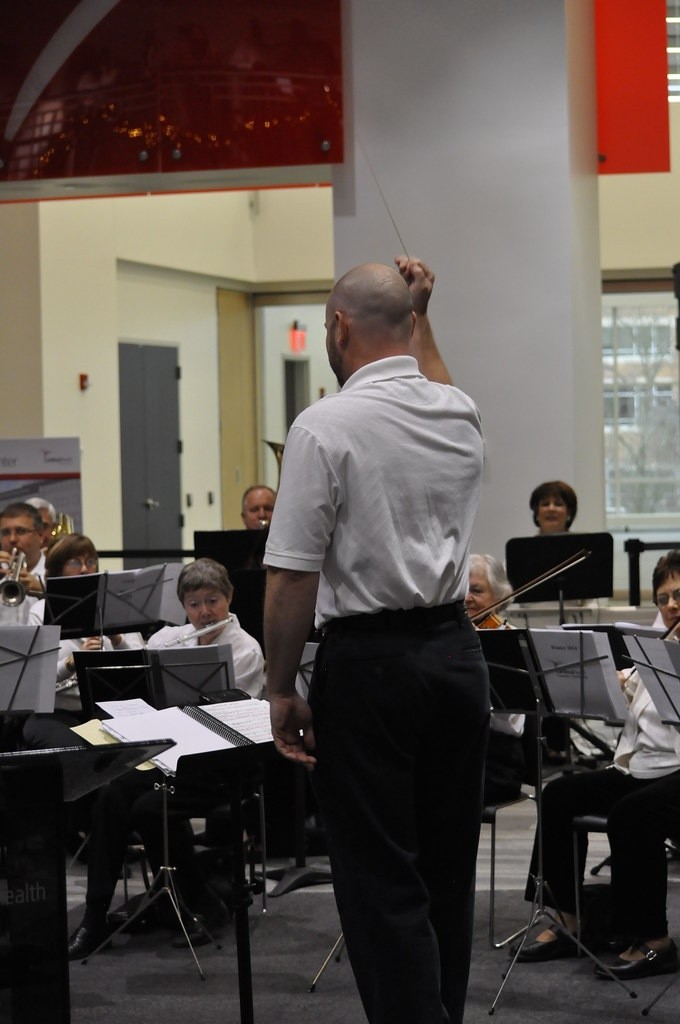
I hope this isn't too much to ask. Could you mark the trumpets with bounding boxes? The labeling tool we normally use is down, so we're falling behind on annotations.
[1,546,28,607]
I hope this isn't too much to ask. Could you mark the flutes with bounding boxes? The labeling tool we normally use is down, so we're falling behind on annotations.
[154,617,235,648]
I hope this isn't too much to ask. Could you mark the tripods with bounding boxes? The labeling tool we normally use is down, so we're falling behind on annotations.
[269,757,335,902]
[479,631,638,1016]
[83,655,229,982]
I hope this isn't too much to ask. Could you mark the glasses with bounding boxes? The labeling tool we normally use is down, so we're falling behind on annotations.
[653,588,680,605]
[0,528,39,536]
[65,557,96,568]
[44,524,53,530]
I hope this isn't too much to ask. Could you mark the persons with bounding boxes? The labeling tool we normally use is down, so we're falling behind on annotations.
[0,478,680,979]
[265,257,480,1024]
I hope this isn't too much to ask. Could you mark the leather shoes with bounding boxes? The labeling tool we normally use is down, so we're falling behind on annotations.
[174,877,254,948]
[68,919,112,961]
[509,924,600,962]
[594,938,680,980]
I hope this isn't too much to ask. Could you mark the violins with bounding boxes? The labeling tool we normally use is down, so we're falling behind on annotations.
[473,614,516,629]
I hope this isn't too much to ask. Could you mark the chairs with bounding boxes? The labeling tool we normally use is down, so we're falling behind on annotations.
[112,793,260,933]
[573,816,613,896]
[466,628,547,949]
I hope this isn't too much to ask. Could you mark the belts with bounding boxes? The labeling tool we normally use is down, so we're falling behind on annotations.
[316,599,468,634]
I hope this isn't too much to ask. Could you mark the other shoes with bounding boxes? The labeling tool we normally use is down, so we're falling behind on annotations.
[118,848,145,878]
